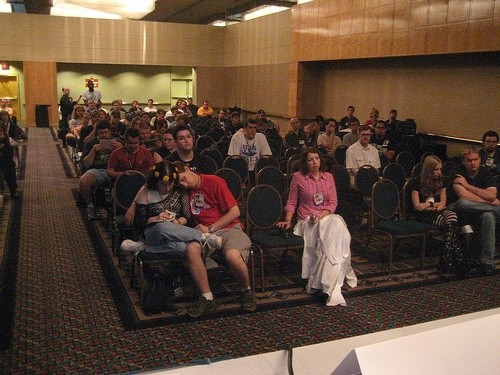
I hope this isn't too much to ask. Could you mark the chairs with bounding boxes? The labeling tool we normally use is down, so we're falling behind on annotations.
[71,115,500,308]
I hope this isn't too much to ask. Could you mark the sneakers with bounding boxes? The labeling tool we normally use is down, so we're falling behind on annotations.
[202,232,223,251]
[186,295,216,318]
[120,239,145,256]
[87,205,97,221]
[239,289,256,312]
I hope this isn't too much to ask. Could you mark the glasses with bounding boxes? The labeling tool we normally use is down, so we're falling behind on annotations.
[360,134,371,137]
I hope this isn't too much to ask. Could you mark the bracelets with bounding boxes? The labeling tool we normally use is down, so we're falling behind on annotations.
[208,225,214,233]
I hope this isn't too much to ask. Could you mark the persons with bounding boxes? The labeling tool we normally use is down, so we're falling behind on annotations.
[163,126,213,175]
[124,162,256,316]
[228,119,272,171]
[138,120,162,154]
[412,156,468,278]
[449,151,500,276]
[288,106,399,226]
[216,105,275,133]
[0,99,22,206]
[80,121,123,221]
[276,147,357,308]
[120,166,222,253]
[108,129,153,177]
[478,130,500,175]
[59,81,212,134]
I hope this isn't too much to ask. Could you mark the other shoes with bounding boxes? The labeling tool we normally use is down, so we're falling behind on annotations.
[442,266,457,279]
[482,264,494,275]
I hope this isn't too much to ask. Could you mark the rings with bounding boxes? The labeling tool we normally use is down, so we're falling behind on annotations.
[163,218,165,220]
